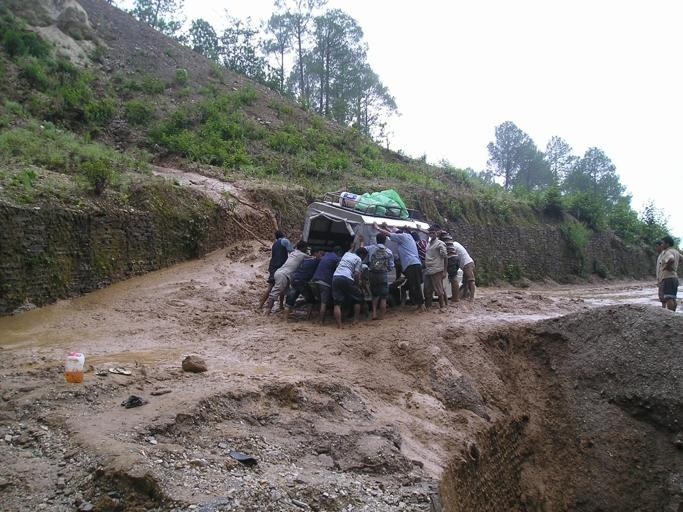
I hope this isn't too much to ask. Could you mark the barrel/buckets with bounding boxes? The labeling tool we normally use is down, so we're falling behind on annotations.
[65,352,85,383]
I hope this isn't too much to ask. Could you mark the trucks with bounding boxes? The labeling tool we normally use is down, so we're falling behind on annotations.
[301,192,476,308]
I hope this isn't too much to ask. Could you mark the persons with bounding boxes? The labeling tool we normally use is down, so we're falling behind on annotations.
[253,220,477,331]
[658,234,683,313]
[653,241,673,308]
